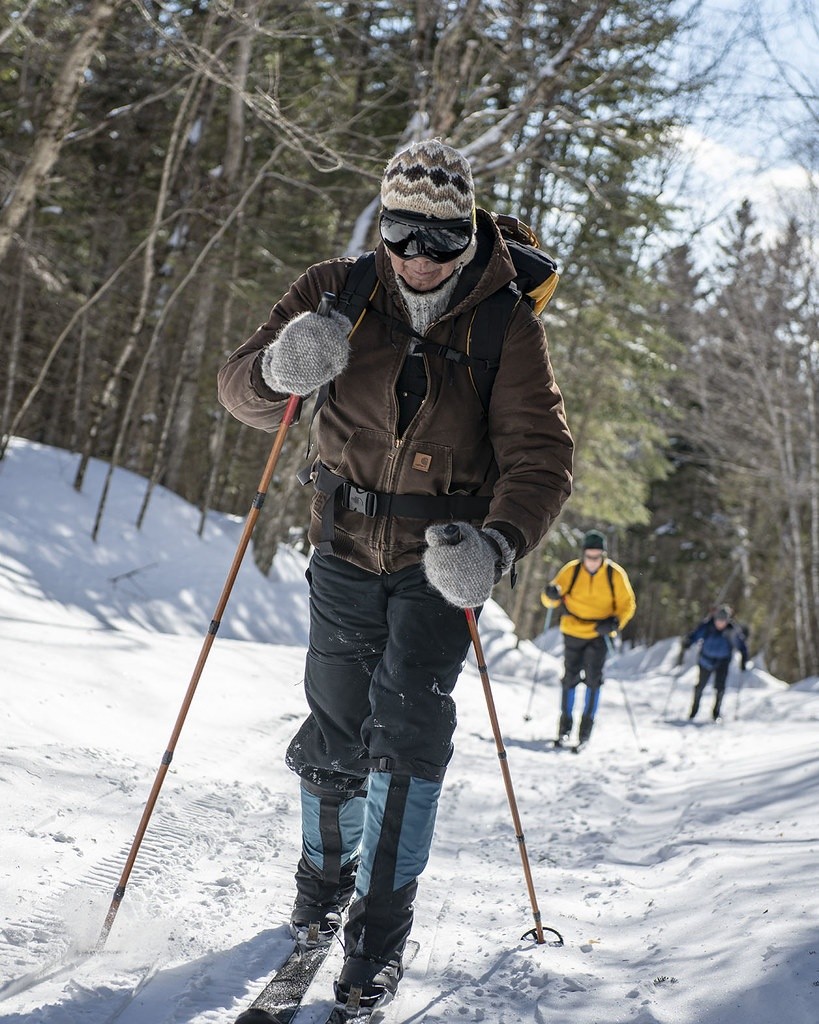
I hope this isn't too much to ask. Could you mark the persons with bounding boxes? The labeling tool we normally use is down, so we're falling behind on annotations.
[681,603,750,721]
[217,140,574,1013]
[541,531,637,744]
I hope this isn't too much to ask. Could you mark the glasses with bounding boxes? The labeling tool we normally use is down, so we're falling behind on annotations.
[379,209,473,264]
[584,553,601,560]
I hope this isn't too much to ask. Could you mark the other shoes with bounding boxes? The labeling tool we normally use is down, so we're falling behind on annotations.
[291,901,345,943]
[336,951,401,1009]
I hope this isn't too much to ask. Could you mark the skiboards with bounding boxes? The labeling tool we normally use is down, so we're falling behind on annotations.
[234,939,335,1024]
[324,938,421,1024]
[554,736,588,754]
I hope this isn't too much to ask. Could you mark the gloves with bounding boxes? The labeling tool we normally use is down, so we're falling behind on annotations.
[595,615,620,637]
[545,583,561,601]
[262,309,353,396]
[682,637,691,649]
[423,521,515,608]
[741,660,747,672]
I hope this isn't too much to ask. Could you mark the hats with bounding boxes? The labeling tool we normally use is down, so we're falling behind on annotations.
[582,530,607,551]
[714,605,731,620]
[381,139,473,220]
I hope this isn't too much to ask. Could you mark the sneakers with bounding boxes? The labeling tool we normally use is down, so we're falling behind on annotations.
[559,715,573,734]
[579,717,594,741]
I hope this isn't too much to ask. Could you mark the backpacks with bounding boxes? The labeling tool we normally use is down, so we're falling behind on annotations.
[558,560,616,625]
[295,210,559,556]
